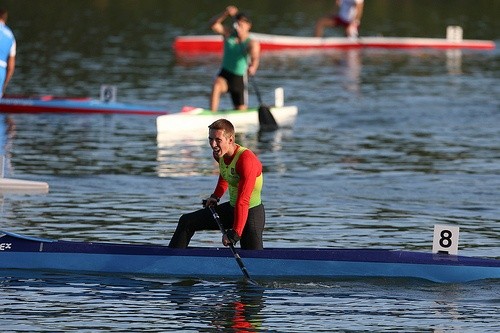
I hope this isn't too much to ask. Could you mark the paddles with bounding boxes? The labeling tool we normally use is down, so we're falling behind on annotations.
[231,14,279,132]
[201,197,262,287]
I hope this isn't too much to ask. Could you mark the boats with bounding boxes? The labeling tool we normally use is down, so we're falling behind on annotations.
[156,86,298,135]
[0,84,166,121]
[173,25,496,58]
[0,229,500,284]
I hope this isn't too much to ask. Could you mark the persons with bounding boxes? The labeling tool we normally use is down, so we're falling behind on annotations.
[210,5,260,111]
[316,0,364,37]
[168,119,265,248]
[0,7,16,98]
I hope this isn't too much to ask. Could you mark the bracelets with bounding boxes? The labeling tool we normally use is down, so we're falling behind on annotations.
[210,194,220,202]
